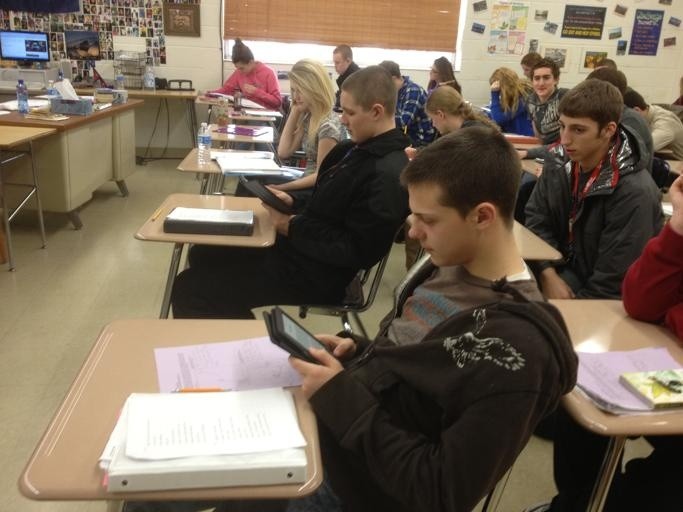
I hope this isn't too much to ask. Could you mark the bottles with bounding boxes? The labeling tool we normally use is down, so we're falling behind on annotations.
[47,79,57,113]
[233,88,242,111]
[144,65,155,91]
[217,96,229,128]
[115,70,124,90]
[57,67,64,81]
[196,122,211,164]
[16,79,29,114]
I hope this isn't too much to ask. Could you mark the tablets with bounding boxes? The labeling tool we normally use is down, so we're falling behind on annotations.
[238,173,290,213]
[272,307,336,365]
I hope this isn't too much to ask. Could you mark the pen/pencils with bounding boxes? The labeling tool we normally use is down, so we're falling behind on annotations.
[151,209,163,221]
[171,387,231,392]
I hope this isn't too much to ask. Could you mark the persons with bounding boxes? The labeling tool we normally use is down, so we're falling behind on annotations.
[532,173,683,512]
[206,38,282,110]
[235,59,347,200]
[171,64,414,323]
[333,46,683,170]
[288,125,579,512]
[521,79,662,298]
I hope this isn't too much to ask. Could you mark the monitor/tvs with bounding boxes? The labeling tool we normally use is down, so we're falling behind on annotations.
[0,30,50,68]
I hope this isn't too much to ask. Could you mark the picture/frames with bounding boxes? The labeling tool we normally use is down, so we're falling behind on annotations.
[162,2,201,37]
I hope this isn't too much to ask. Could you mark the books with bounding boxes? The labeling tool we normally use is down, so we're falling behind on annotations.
[99,393,308,494]
[164,207,254,237]
